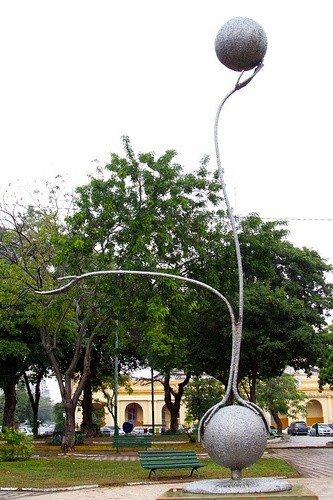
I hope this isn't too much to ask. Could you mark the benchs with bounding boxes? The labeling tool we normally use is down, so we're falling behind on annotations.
[112,435,153,454]
[138,449,207,480]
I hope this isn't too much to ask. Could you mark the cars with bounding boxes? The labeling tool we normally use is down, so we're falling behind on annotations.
[270,425,276,433]
[310,423,333,436]
[0,423,56,437]
[100,423,195,436]
[286,421,308,436]
